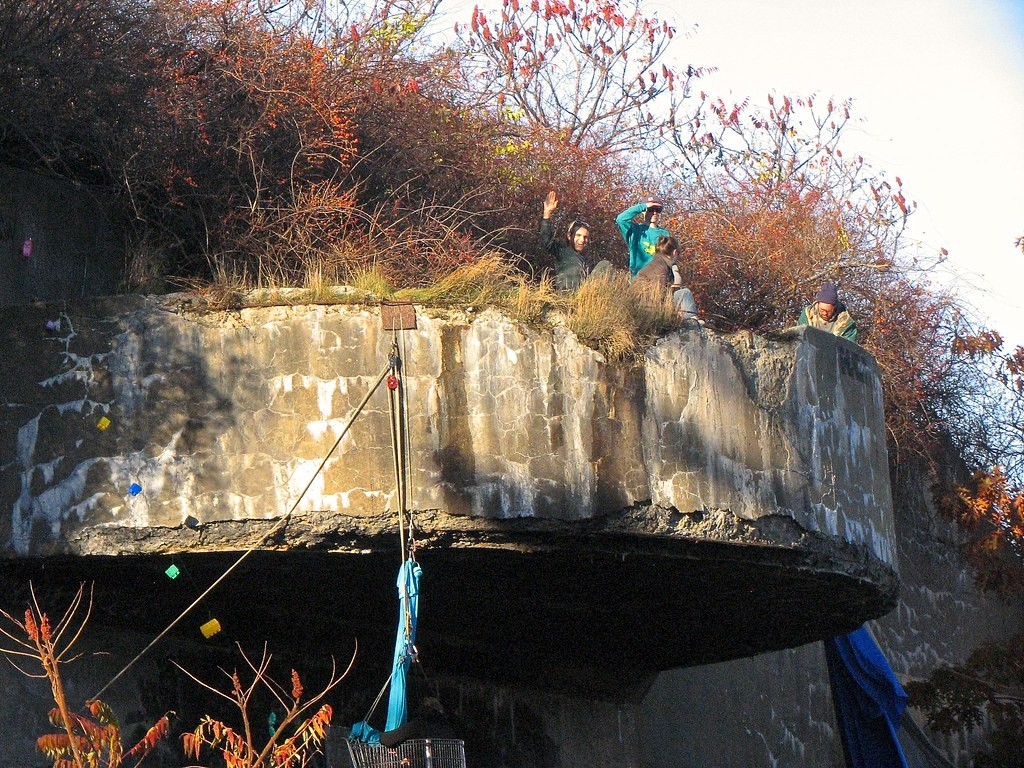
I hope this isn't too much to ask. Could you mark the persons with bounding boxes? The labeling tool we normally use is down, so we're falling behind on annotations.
[629,235,705,327]
[541,191,591,298]
[798,283,858,343]
[615,195,678,284]
[269,693,318,768]
[379,698,458,768]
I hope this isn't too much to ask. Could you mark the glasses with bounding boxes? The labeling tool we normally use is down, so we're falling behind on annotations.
[568,220,590,233]
[648,207,662,212]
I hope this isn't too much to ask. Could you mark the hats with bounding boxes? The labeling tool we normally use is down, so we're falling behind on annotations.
[642,195,663,205]
[817,283,837,304]
